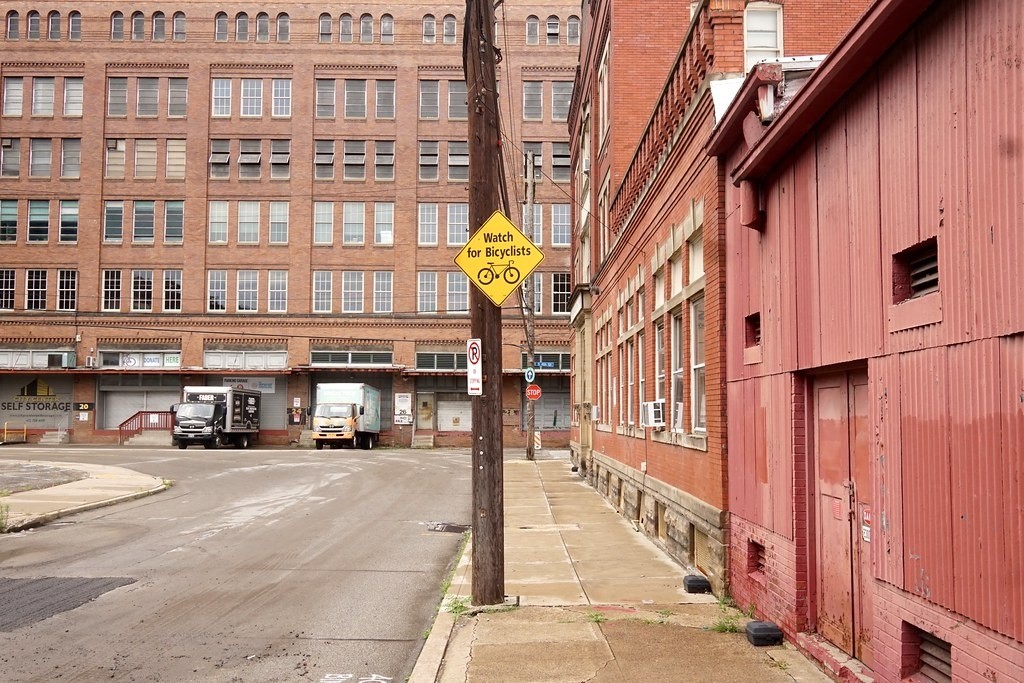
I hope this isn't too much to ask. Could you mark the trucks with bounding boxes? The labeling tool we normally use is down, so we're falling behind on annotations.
[169,385,261,449]
[307,382,382,450]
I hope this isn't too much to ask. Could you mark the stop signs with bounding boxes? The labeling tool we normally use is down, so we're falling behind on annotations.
[526,384,542,400]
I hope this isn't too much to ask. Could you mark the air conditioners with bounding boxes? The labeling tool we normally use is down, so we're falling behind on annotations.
[47,353,76,367]
[591,406,600,421]
[86,356,100,369]
[641,402,666,427]
